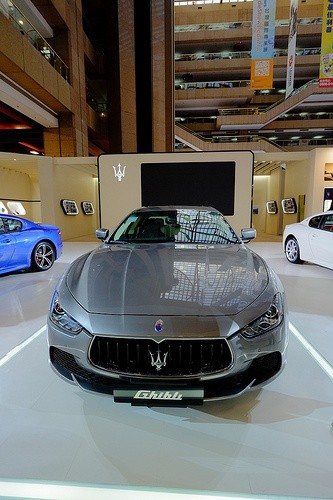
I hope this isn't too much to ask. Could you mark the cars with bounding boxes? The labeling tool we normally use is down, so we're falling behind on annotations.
[0,214,64,276]
[45,205,289,408]
[282,211,333,270]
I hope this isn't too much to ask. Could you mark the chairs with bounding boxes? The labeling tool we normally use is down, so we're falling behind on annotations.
[142,218,164,239]
